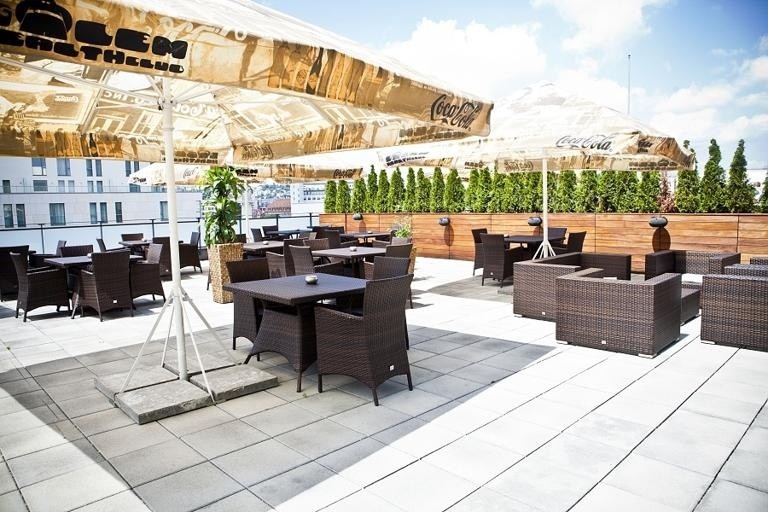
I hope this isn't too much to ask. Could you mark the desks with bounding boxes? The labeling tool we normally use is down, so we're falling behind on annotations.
[221,273,371,392]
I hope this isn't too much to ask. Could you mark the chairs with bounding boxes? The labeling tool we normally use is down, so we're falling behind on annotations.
[2,231,201,322]
[314,274,415,405]
[471,227,587,289]
[227,226,414,361]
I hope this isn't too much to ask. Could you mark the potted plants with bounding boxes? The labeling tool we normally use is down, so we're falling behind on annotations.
[202,168,244,303]
[318,137,768,273]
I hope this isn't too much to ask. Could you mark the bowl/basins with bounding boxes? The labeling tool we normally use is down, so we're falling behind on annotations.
[305,276,318,285]
[349,246,358,252]
[263,241,269,245]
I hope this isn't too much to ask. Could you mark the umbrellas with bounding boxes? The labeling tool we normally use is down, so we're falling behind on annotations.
[127,155,369,242]
[0,0,494,383]
[376,79,696,262]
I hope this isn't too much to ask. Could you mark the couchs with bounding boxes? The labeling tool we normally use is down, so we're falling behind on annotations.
[514,251,768,359]
